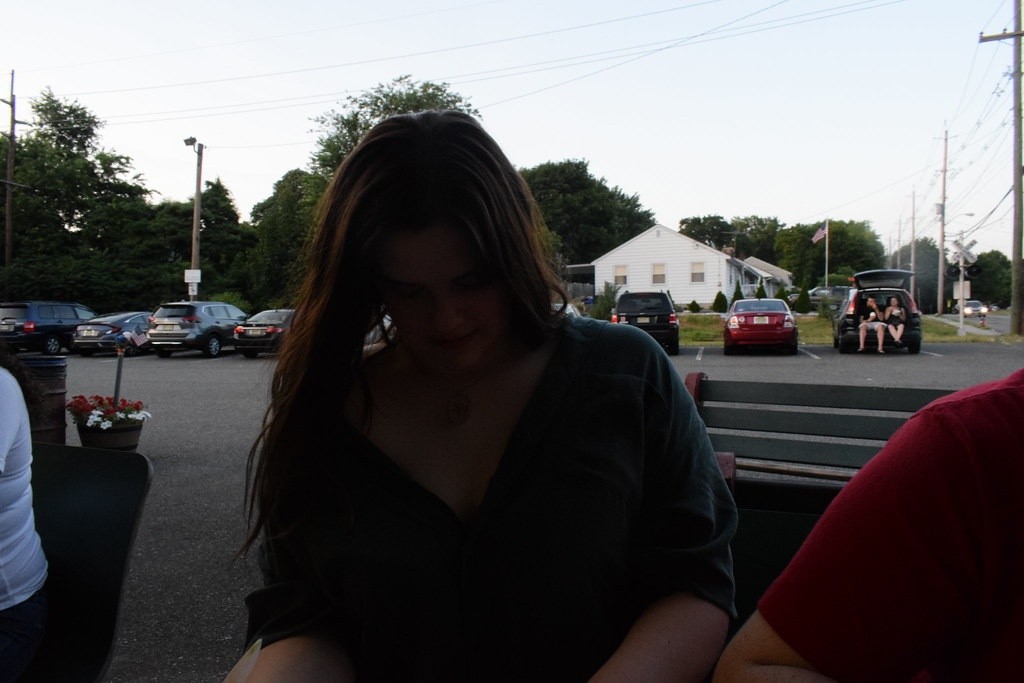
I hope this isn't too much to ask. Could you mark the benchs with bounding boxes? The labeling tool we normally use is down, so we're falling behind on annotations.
[684,371,960,640]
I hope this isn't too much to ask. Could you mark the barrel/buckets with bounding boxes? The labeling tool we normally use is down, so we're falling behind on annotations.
[2,355,68,448]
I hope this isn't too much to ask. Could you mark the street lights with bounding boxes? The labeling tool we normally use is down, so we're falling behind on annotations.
[936,212,976,315]
[184,136,207,300]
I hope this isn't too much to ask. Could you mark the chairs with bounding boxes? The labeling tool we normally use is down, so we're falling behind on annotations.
[16,441,152,681]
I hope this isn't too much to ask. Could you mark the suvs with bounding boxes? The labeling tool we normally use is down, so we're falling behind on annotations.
[830,268,923,355]
[146,299,251,358]
[0,300,100,355]
[610,289,680,356]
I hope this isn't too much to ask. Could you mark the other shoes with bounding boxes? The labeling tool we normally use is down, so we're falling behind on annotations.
[893,341,905,348]
[857,348,865,352]
[877,349,885,353]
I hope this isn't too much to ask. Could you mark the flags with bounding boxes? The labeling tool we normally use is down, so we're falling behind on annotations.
[131,325,147,346]
[811,222,826,243]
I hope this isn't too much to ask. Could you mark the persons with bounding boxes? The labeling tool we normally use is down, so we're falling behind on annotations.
[225,110,739,683]
[0,347,48,683]
[857,294,886,353]
[885,296,906,343]
[713,366,1024,683]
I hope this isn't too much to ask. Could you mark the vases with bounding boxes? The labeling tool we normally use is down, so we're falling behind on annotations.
[77,414,142,454]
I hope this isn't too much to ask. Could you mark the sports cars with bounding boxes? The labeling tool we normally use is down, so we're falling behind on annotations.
[71,310,156,358]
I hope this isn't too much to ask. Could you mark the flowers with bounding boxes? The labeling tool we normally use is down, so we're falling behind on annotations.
[70,393,153,430]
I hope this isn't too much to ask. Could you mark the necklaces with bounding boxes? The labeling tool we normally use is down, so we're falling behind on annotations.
[419,361,474,423]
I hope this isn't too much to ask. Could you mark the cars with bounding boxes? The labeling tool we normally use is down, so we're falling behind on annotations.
[553,302,582,320]
[788,286,846,313]
[951,299,1001,318]
[720,298,798,356]
[233,309,297,358]
